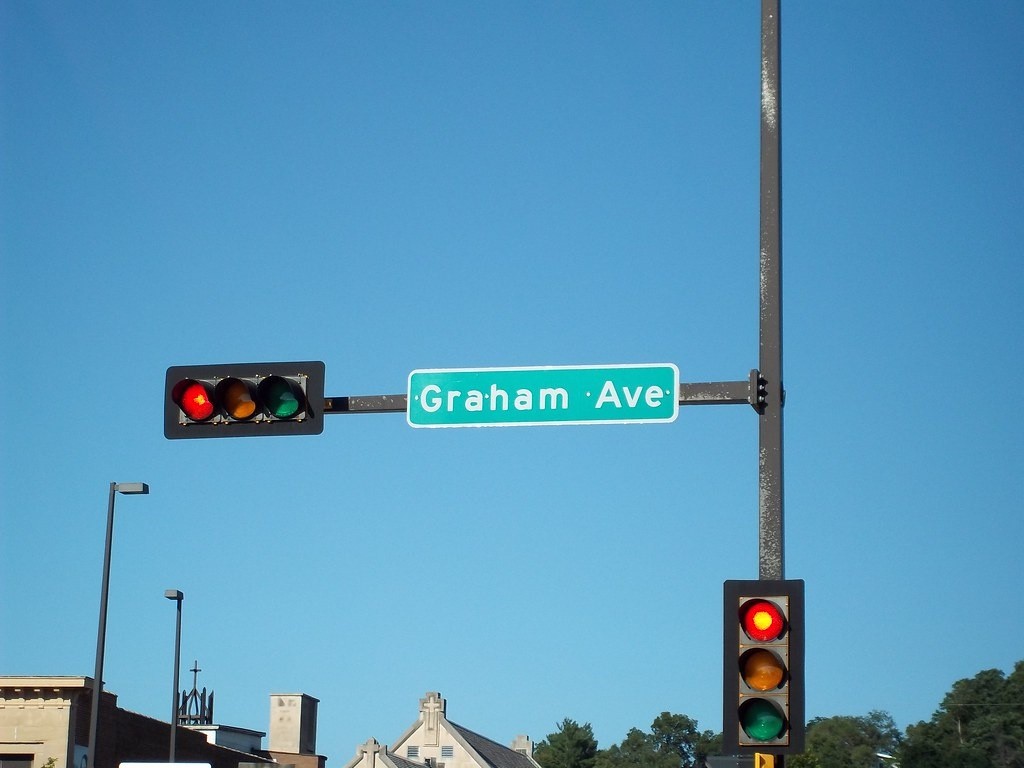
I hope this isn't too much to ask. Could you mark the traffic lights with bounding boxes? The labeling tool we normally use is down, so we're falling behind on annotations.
[724,579,807,755]
[163,361,325,438]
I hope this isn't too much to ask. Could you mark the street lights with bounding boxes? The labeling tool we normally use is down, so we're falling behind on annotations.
[85,480,150,768]
[164,589,185,762]
